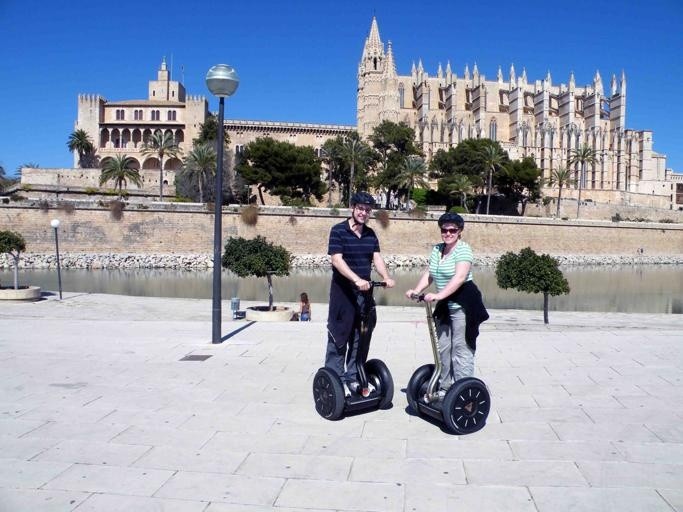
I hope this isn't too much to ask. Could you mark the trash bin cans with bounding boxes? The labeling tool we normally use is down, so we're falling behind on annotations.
[231,298,240,319]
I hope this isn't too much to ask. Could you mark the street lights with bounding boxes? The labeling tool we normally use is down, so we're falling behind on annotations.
[199,60,239,342]
[50,218,63,298]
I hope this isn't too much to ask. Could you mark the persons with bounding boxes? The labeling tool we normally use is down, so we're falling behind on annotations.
[295,292,311,322]
[406,210,489,402]
[323,191,395,395]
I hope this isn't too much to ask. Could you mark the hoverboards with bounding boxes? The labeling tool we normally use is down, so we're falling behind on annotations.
[310,280,396,421]
[406,289,493,433]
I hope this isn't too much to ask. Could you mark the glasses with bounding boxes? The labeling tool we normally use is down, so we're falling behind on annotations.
[358,207,372,214]
[441,227,460,234]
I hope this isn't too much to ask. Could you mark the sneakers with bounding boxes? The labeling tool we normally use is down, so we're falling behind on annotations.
[351,382,376,392]
[344,384,352,398]
[437,390,447,397]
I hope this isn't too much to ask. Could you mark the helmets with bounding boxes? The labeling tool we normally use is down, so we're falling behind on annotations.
[438,213,464,228]
[351,192,374,205]
